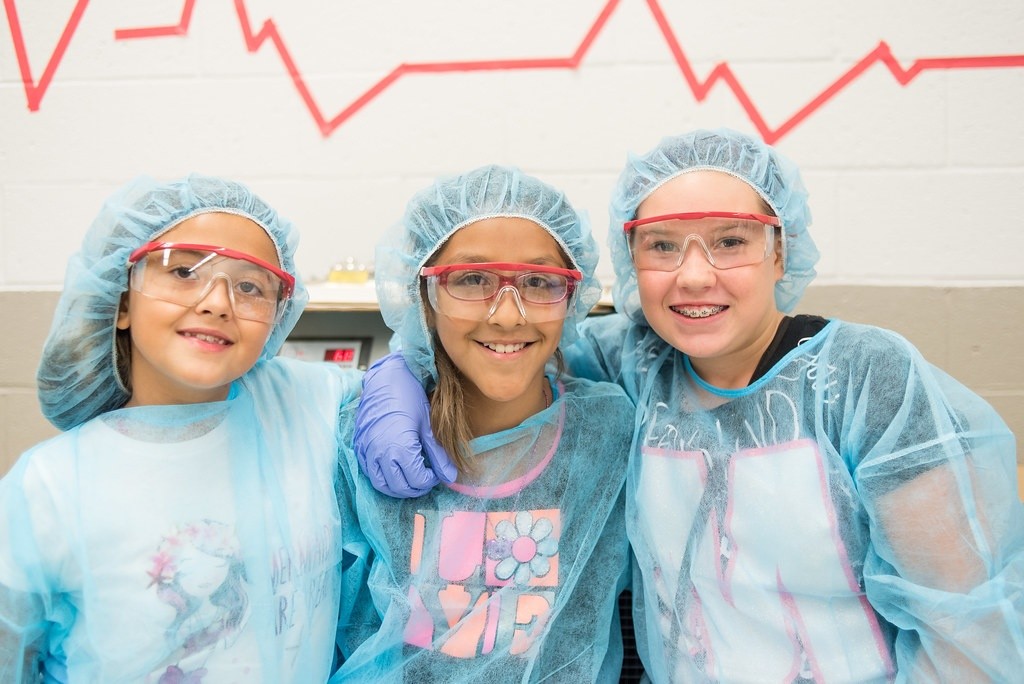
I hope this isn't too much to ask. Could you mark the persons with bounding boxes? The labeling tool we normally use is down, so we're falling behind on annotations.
[0,172,367,684]
[353,126,1024,684]
[331,167,635,684]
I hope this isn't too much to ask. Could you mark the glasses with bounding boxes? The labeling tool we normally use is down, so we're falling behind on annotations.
[419,262,584,323]
[128,241,295,325]
[623,211,780,271]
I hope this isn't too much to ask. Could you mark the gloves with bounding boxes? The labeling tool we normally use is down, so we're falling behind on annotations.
[353,350,457,498]
